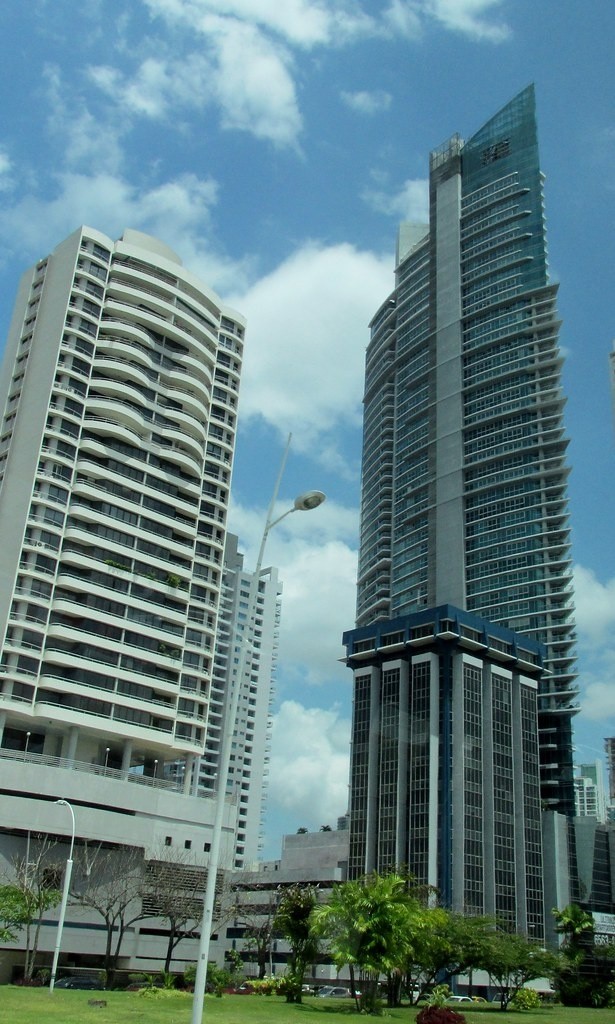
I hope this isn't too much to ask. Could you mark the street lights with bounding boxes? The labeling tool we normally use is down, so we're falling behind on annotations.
[189,428,336,1024]
[47,798,75,989]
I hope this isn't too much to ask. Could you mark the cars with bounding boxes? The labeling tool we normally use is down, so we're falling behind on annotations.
[127,983,161,992]
[216,979,559,1005]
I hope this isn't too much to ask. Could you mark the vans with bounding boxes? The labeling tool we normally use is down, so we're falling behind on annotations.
[55,976,104,992]
